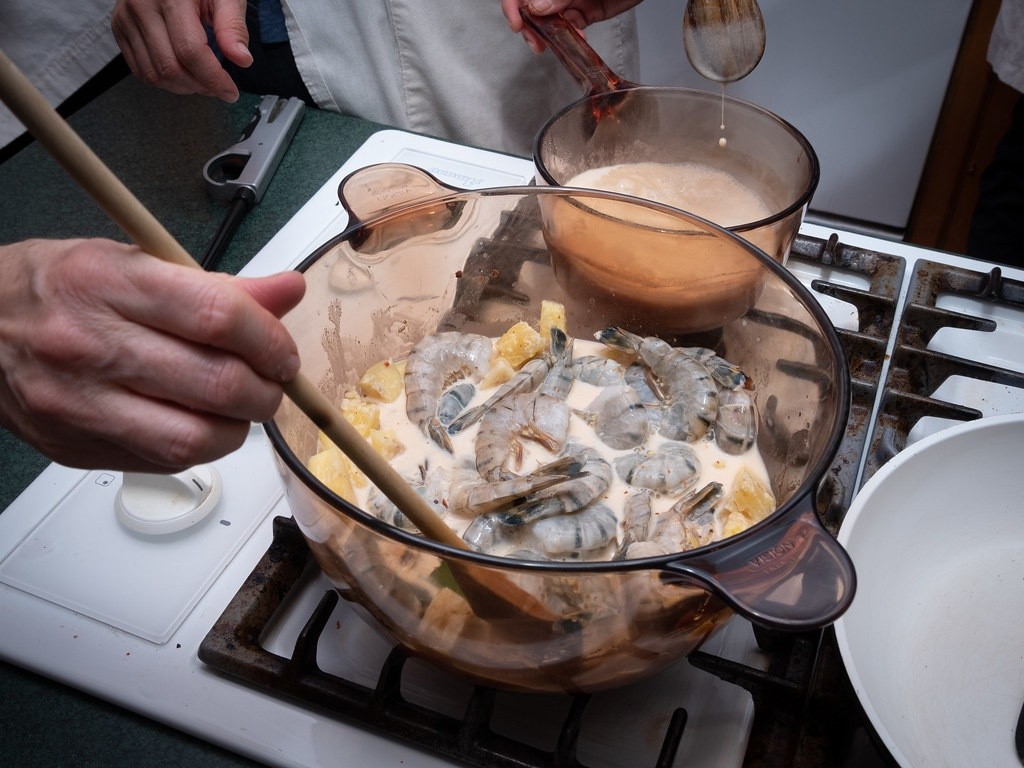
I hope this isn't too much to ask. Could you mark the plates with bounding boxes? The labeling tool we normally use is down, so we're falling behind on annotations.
[832,410,1024,768]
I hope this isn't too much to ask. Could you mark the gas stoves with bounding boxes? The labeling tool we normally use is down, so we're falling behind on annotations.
[0,129,1024,768]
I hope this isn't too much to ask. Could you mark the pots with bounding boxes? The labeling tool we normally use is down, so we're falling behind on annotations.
[518,0,821,331]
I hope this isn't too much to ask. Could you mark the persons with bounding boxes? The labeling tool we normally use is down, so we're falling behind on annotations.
[499,0,1024,277]
[106,0,651,169]
[0,0,311,480]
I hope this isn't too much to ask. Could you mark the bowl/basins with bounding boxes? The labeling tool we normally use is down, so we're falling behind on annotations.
[260,161,857,695]
